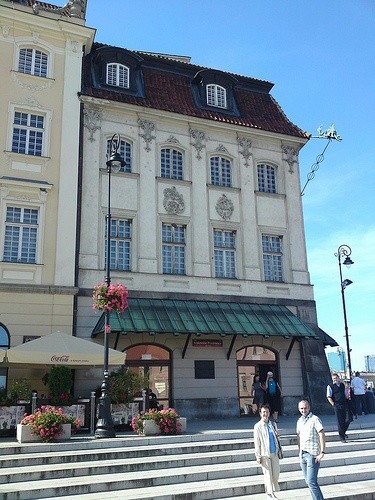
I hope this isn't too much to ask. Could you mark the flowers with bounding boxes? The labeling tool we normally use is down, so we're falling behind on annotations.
[21,405,81,443]
[92,281,129,313]
[132,407,182,436]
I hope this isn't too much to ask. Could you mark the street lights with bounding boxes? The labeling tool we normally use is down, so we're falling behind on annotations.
[94,131,128,438]
[333,244,355,400]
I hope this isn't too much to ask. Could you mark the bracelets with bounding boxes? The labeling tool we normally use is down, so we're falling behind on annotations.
[321,451,325,454]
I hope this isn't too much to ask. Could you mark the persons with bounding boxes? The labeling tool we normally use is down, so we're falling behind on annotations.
[327,373,352,443]
[134,389,161,413]
[351,371,368,415]
[251,375,265,416]
[296,400,326,500]
[365,387,375,414]
[30,390,42,413]
[254,405,283,500]
[263,371,283,423]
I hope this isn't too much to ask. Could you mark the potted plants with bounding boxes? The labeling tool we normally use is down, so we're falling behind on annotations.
[110,366,152,426]
[0,365,86,439]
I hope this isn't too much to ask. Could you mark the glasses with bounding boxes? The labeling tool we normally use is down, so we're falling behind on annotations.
[269,376,273,378]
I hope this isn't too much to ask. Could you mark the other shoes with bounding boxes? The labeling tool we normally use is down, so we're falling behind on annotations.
[341,438,346,442]
[345,433,349,439]
[272,493,279,500]
[272,418,279,423]
[267,493,274,500]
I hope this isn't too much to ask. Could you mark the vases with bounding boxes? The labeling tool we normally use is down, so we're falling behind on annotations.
[16,424,71,444]
[142,417,187,436]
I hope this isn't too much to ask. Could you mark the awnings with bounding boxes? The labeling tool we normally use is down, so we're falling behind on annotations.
[93,296,319,361]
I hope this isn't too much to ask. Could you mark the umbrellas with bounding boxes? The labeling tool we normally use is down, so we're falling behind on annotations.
[5,330,126,364]
[0,348,6,364]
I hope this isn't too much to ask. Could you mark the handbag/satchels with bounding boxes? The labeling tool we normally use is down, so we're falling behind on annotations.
[278,442,284,459]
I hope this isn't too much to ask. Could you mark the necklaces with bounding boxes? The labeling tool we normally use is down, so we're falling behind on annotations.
[269,380,272,385]
